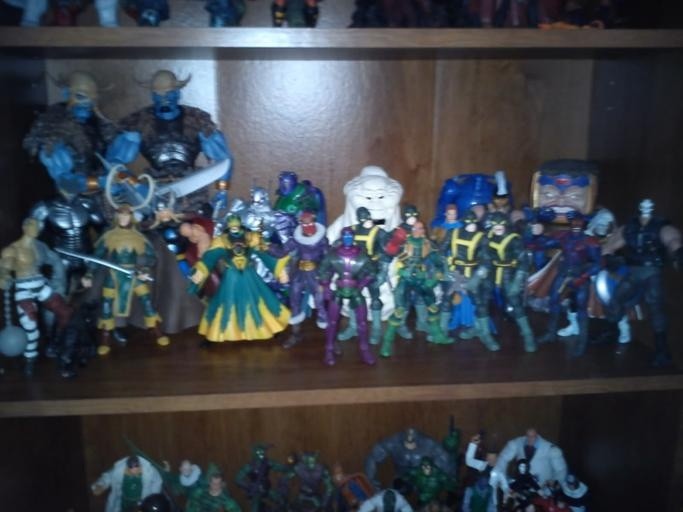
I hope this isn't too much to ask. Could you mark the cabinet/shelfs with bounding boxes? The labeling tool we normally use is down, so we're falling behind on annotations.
[0,19,683,419]
